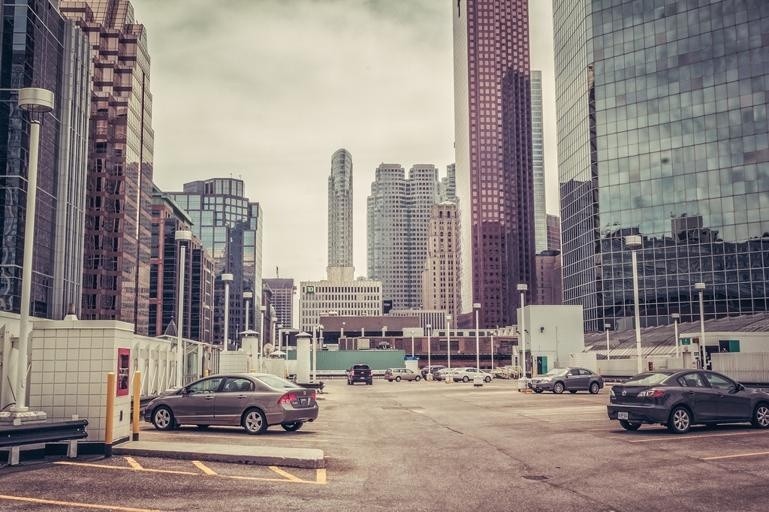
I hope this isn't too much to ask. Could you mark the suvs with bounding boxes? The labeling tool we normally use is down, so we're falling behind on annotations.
[345,364,373,385]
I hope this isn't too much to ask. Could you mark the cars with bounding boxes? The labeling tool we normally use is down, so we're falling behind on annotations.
[420,364,521,383]
[142,371,319,435]
[606,369,769,434]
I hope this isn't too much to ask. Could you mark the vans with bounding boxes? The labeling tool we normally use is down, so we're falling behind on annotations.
[384,368,423,382]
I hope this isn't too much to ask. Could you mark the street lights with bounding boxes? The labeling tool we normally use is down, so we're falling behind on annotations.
[221,271,233,351]
[473,302,484,386]
[516,283,530,394]
[241,290,289,360]
[622,234,644,376]
[311,324,321,381]
[489,329,494,372]
[527,366,603,395]
[693,282,707,370]
[670,312,680,359]
[411,315,458,383]
[5,82,59,421]
[603,323,612,359]
[173,229,194,390]
